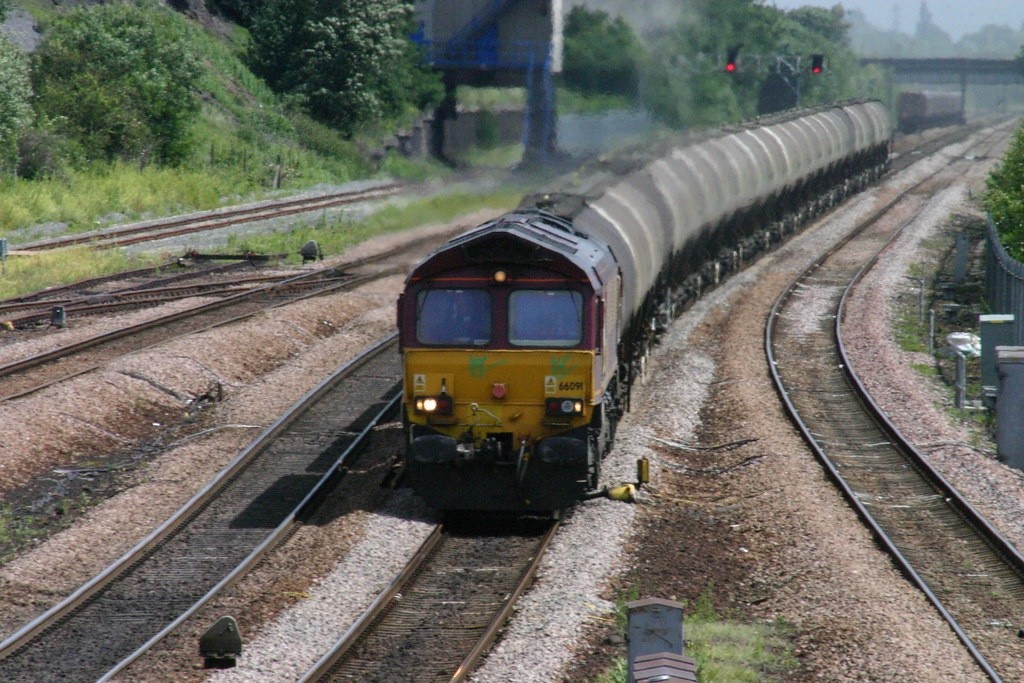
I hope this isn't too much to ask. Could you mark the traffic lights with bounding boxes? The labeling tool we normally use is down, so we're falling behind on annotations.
[813,54,823,74]
[726,48,739,73]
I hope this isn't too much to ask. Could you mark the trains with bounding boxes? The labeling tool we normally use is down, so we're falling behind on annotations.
[897,91,966,129]
[395,98,893,511]
